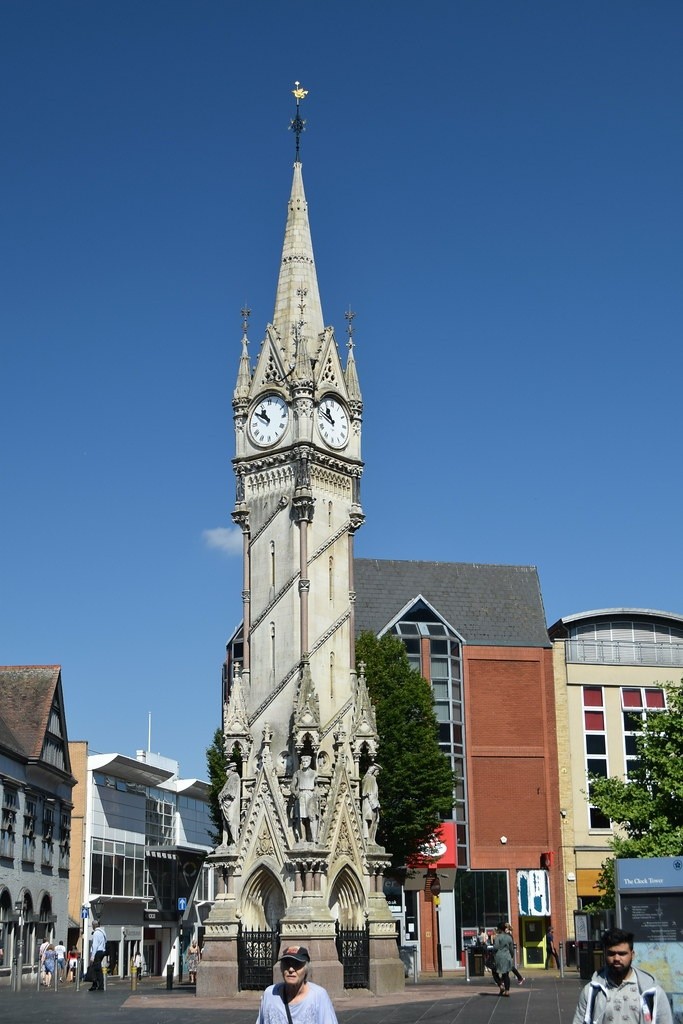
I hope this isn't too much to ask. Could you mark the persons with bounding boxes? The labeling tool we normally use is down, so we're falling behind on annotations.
[255,945,339,1024]
[185,939,204,983]
[475,922,562,997]
[40,920,143,992]
[572,926,673,1024]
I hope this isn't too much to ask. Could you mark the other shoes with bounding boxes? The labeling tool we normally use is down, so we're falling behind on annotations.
[97,987,104,991]
[517,977,525,986]
[60,977,63,983]
[498,989,505,996]
[88,987,97,991]
[503,994,510,997]
[494,980,504,986]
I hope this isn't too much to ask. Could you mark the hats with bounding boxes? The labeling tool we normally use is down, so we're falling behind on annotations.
[278,946,310,963]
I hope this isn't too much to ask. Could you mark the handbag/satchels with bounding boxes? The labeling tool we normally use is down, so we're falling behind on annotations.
[485,955,496,969]
[83,966,94,982]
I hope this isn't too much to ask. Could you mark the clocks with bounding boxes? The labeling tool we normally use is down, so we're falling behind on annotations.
[315,396,351,450]
[246,393,289,450]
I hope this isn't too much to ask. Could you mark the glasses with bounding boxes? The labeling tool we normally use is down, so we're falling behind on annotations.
[281,960,305,970]
